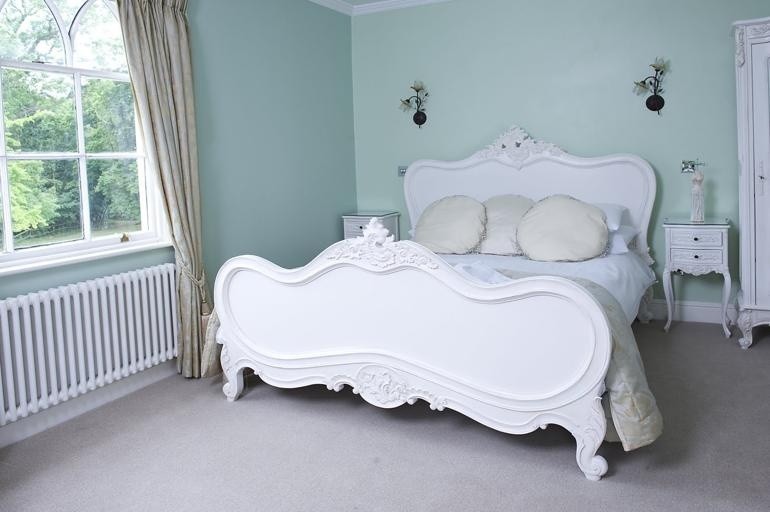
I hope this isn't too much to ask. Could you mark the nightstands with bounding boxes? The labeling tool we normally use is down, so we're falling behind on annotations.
[342,211,401,243]
[661,216,731,338]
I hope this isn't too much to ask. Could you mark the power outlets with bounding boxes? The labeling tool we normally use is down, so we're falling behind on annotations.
[398,166,408,176]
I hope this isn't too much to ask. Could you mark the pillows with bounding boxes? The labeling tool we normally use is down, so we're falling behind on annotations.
[608,225,641,254]
[474,194,533,256]
[592,201,626,233]
[517,193,608,262]
[414,195,487,256]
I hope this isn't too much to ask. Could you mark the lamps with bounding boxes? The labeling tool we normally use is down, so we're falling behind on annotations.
[633,57,668,111]
[399,81,429,128]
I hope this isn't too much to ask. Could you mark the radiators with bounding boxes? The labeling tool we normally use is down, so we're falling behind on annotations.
[1,263,180,427]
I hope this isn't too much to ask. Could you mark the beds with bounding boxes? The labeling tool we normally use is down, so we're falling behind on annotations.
[214,125,664,481]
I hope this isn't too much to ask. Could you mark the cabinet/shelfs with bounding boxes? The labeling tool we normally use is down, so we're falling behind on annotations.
[750,42,770,309]
